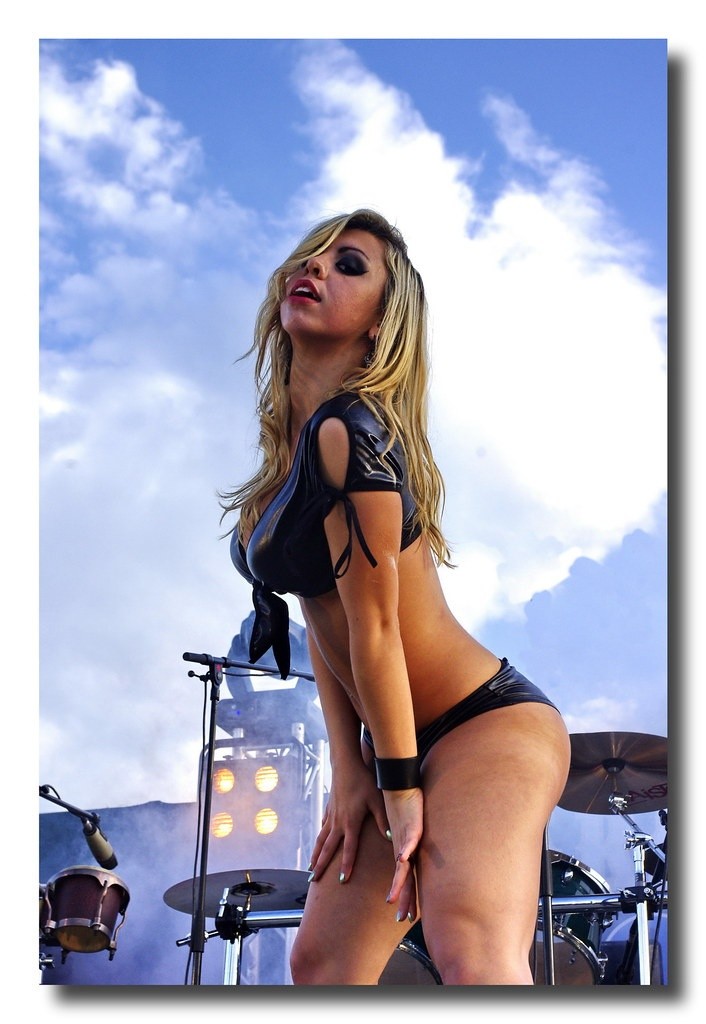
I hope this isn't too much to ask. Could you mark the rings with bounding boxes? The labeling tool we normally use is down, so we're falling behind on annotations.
[395,856,399,863]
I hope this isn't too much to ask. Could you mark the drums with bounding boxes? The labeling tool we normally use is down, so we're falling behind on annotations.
[528,849,614,985]
[378,919,441,985]
[39,865,130,953]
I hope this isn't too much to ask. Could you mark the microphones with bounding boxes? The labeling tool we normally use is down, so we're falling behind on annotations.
[81,819,118,871]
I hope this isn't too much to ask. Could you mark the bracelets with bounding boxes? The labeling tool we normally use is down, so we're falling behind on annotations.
[375,754,421,791]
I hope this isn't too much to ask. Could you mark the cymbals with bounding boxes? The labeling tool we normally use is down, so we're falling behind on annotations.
[554,730,668,815]
[162,868,312,919]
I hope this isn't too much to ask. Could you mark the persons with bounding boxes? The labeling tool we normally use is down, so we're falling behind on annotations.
[220,209,571,986]
